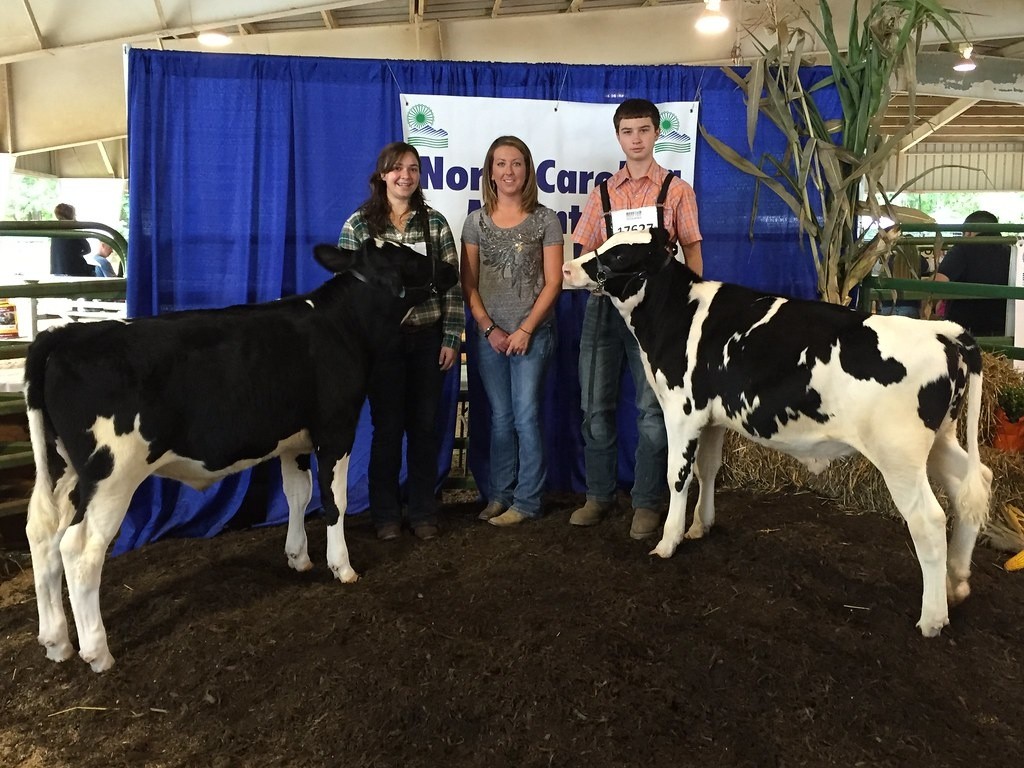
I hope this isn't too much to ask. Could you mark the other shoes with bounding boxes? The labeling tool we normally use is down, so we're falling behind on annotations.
[377,522,401,540]
[478,501,506,521]
[489,505,530,526]
[417,522,438,539]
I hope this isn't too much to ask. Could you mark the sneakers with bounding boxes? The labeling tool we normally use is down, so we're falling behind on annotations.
[569,494,622,526]
[630,504,661,540]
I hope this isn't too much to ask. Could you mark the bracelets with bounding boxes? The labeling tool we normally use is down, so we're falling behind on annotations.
[520,328,532,335]
[484,323,498,339]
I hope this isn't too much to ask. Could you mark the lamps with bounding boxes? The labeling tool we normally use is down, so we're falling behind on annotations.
[694,0,729,35]
[197,27,232,47]
[953,43,976,72]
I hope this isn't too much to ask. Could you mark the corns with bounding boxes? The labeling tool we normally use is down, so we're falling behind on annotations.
[842,223,902,291]
[1004,549,1024,571]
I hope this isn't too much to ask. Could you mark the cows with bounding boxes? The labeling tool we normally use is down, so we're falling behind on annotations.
[26,240,463,676]
[561,226,994,638]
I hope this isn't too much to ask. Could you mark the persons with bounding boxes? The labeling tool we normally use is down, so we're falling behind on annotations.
[50,203,118,312]
[874,211,1011,337]
[569,98,704,539]
[334,142,465,539]
[95,241,123,277]
[460,135,564,527]
